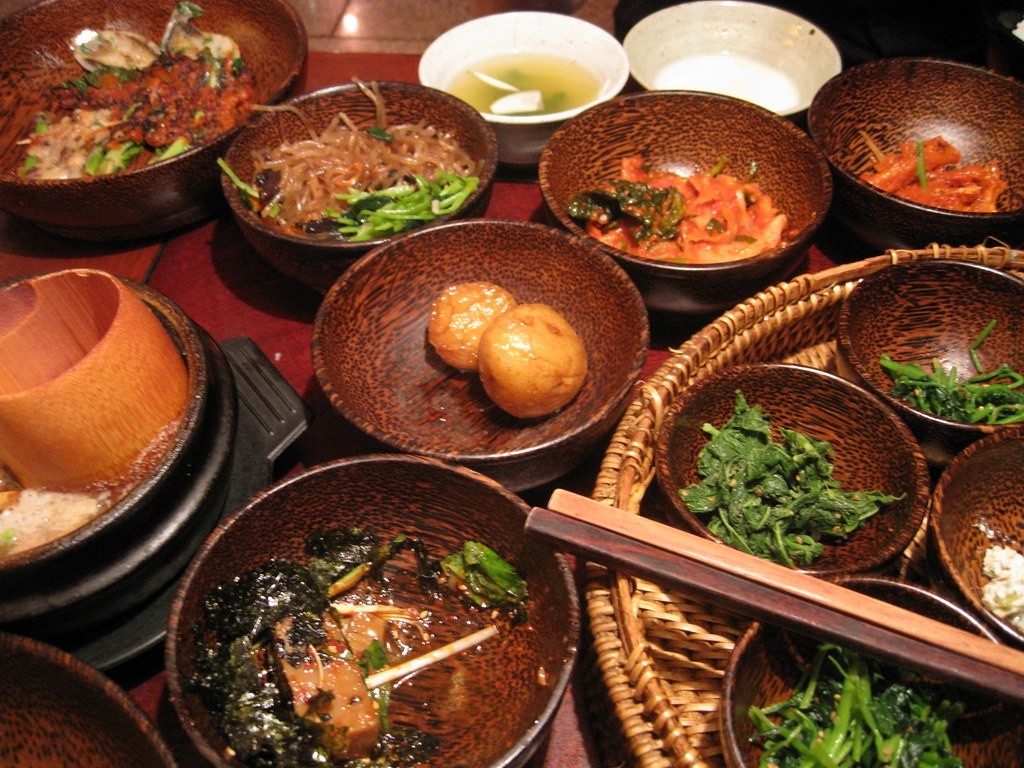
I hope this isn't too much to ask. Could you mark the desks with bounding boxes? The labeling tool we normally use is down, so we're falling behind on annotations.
[0,52,852,768]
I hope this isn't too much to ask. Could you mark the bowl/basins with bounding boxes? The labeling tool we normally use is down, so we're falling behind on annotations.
[808,59,1024,250]
[538,89,833,311]
[837,257,1024,463]
[932,429,1024,643]
[166,454,580,768]
[720,576,1002,768]
[656,362,929,577]
[0,0,307,244]
[0,634,179,768]
[222,80,499,286]
[0,323,238,638]
[418,12,630,165]
[623,0,842,116]
[0,270,206,568]
[311,218,649,492]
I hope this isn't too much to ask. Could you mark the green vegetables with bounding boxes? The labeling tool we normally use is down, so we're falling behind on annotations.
[679,387,911,572]
[749,635,974,768]
[181,524,529,768]
[20,47,242,174]
[214,125,481,242]
[881,318,1024,427]
[565,153,730,246]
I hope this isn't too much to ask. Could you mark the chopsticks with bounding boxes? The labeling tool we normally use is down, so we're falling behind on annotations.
[524,488,1024,700]
